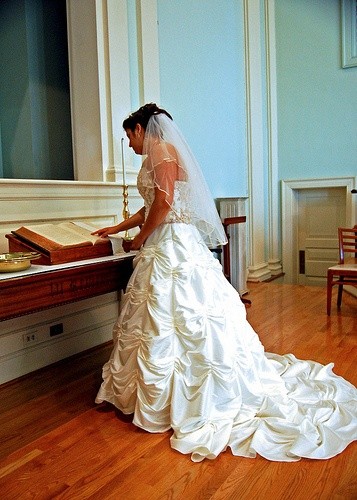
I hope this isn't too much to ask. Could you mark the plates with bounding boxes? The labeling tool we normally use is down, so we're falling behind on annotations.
[0,252,42,272]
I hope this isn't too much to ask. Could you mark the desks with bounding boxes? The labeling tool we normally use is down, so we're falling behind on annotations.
[0,251,136,322]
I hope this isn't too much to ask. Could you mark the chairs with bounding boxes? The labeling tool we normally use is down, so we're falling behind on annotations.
[326,228,357,316]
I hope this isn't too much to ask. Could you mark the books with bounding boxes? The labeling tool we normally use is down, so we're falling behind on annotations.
[10,222,123,252]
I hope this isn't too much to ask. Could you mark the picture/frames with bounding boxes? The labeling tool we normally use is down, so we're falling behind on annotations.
[338,0,357,69]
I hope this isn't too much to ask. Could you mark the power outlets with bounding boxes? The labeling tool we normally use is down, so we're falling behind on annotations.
[23,330,39,345]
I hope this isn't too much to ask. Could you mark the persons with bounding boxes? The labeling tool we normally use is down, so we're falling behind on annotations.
[91,102,245,434]
